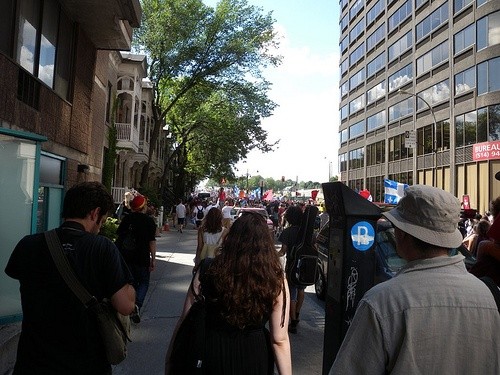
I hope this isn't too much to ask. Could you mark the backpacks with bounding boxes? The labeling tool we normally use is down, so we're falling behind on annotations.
[196,206,204,219]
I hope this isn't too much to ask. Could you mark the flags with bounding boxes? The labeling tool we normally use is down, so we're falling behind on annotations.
[384,179,409,204]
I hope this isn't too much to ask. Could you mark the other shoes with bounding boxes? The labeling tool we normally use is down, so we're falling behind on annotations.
[180,229,183,234]
[288,324,297,333]
[132,304,140,323]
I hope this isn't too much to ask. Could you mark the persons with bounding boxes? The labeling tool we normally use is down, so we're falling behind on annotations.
[165,213,292,375]
[277,207,317,334]
[328,184,500,375]
[3,182,136,375]
[463,197,500,313]
[218,187,225,207]
[176,199,187,233]
[195,207,228,263]
[116,194,157,325]
[189,197,236,228]
[236,199,328,238]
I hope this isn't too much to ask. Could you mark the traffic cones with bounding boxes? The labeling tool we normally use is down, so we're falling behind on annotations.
[163,222,170,231]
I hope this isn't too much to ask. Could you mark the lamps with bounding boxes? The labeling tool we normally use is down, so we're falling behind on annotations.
[77,164,90,175]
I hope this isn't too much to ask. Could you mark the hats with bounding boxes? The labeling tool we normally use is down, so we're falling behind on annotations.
[129,195,146,210]
[379,185,463,248]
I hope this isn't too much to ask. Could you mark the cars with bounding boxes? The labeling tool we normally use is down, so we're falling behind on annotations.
[313,203,408,301]
[233,207,274,233]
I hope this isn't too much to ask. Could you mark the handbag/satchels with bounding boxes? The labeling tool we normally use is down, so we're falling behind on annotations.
[95,300,133,364]
[169,258,210,375]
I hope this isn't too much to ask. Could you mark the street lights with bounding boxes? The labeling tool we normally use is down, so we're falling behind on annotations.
[397,89,439,187]
